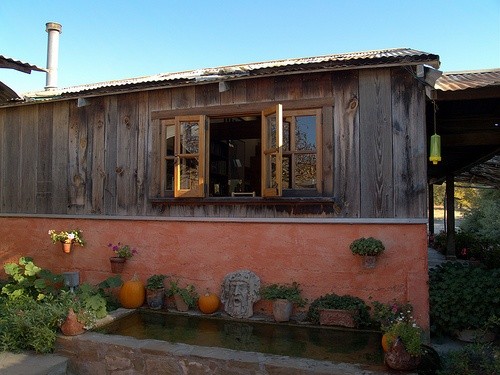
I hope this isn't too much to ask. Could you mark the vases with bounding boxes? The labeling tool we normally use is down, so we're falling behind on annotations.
[109,257,125,274]
[382,332,419,371]
[63,244,71,254]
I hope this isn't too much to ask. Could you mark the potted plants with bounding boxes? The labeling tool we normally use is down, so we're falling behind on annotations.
[164,279,200,312]
[0,256,108,356]
[349,237,385,272]
[144,274,168,311]
[254,281,308,322]
[308,294,372,328]
[426,260,500,344]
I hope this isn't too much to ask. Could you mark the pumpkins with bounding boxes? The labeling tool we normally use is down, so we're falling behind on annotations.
[382,334,396,352]
[119,271,146,308]
[199,288,219,313]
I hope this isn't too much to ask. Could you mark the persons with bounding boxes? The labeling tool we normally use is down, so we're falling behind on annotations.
[221,270,260,318]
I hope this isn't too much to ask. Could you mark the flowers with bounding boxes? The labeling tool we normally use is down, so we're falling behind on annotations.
[108,240,141,258]
[48,227,87,248]
[368,296,428,357]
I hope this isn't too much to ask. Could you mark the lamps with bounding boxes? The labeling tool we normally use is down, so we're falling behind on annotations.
[241,117,257,121]
[429,101,442,165]
